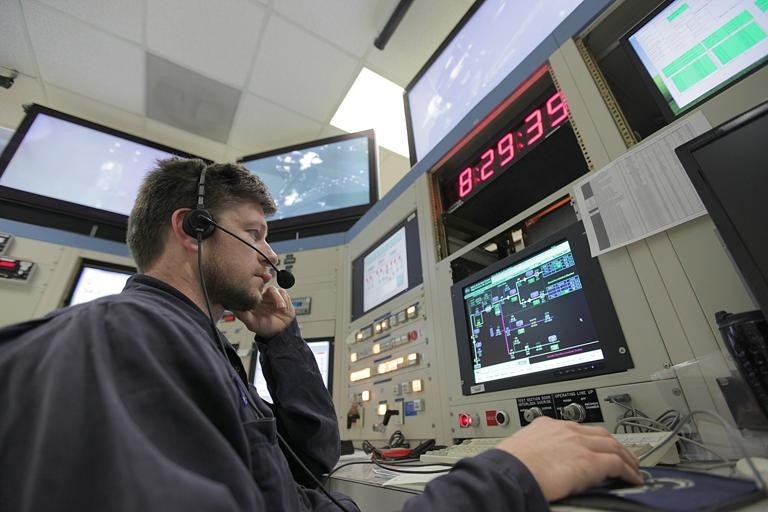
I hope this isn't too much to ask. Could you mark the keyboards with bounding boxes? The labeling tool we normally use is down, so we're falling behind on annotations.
[420,430,681,468]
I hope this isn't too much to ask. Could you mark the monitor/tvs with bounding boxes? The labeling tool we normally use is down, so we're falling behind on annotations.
[351,209,424,321]
[402,0,616,169]
[449,219,635,396]
[619,1,767,125]
[674,102,768,313]
[0,102,214,230]
[236,128,379,242]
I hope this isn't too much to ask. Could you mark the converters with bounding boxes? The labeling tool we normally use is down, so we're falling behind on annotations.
[181,164,215,239]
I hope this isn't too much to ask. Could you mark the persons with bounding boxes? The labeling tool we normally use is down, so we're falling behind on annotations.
[0,156,645,512]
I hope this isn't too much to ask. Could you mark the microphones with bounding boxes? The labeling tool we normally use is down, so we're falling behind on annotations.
[277,270,295,289]
